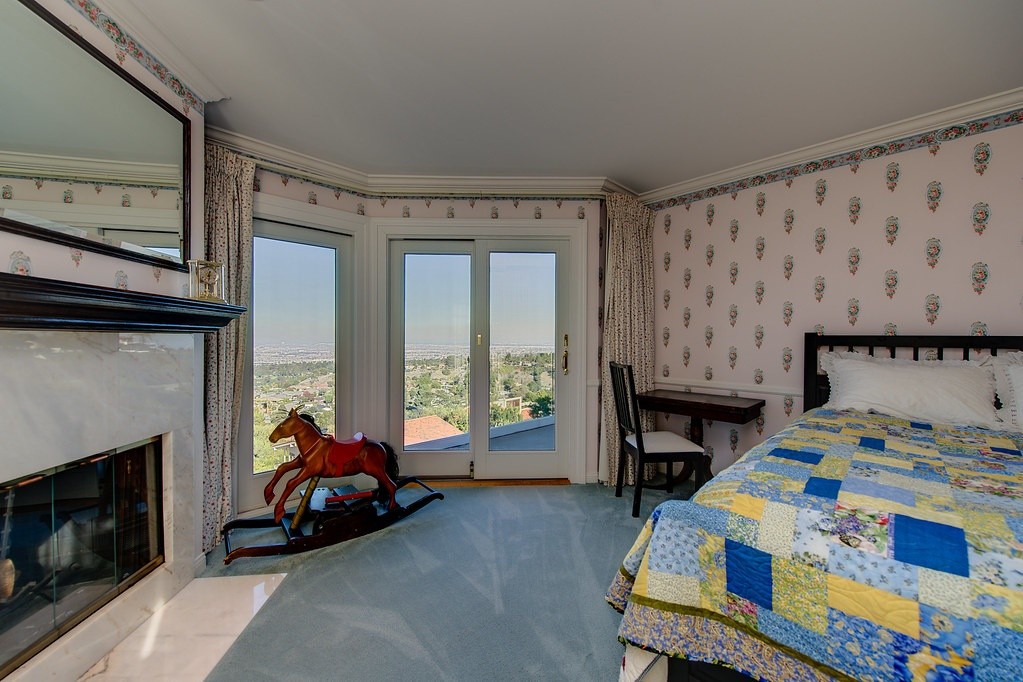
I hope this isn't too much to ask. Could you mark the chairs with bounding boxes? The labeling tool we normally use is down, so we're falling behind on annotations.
[608,360,704,518]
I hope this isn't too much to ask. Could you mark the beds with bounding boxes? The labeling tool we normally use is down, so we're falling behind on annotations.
[602,333,1023,682]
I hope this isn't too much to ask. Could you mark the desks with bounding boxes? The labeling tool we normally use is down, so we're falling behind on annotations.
[636,388,767,496]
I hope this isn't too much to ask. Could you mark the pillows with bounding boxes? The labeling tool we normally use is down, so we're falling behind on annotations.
[820,353,1002,431]
[992,351,1023,433]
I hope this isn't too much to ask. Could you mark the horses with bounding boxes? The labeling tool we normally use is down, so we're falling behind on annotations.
[264,404,399,523]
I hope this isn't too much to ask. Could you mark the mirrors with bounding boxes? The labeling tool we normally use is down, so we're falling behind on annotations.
[0,0,192,273]
